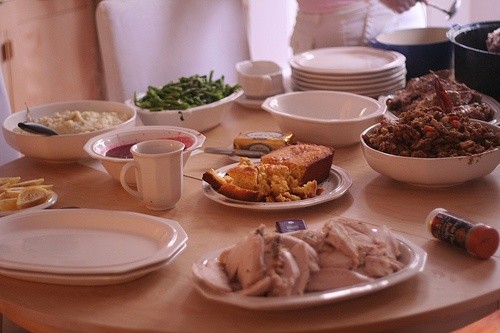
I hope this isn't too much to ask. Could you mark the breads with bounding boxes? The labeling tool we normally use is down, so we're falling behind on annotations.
[201,142,336,200]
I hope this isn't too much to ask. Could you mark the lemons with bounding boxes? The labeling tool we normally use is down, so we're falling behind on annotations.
[0,177,54,211]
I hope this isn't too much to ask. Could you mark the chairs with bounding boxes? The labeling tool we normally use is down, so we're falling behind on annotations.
[94,0,251,104]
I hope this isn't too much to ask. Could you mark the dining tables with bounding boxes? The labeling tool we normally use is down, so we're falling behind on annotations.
[0,101,500,332]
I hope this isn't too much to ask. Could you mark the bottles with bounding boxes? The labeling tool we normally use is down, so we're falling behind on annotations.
[425,206,500,260]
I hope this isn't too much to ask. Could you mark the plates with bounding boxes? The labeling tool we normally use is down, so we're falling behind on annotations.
[203,158,354,209]
[191,224,429,309]
[0,187,58,218]
[290,46,500,125]
[235,93,276,109]
[0,207,189,285]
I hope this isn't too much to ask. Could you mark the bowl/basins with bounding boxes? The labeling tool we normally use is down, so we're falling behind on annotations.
[82,123,205,186]
[128,84,246,132]
[261,89,387,146]
[2,99,137,164]
[361,119,499,187]
[446,19,500,105]
[368,26,459,83]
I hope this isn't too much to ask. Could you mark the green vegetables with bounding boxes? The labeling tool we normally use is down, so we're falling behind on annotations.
[134,71,241,111]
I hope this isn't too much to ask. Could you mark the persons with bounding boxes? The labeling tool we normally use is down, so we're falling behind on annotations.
[291,0,430,59]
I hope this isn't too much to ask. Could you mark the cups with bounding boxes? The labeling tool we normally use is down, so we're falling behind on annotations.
[119,139,185,210]
[234,60,284,100]
